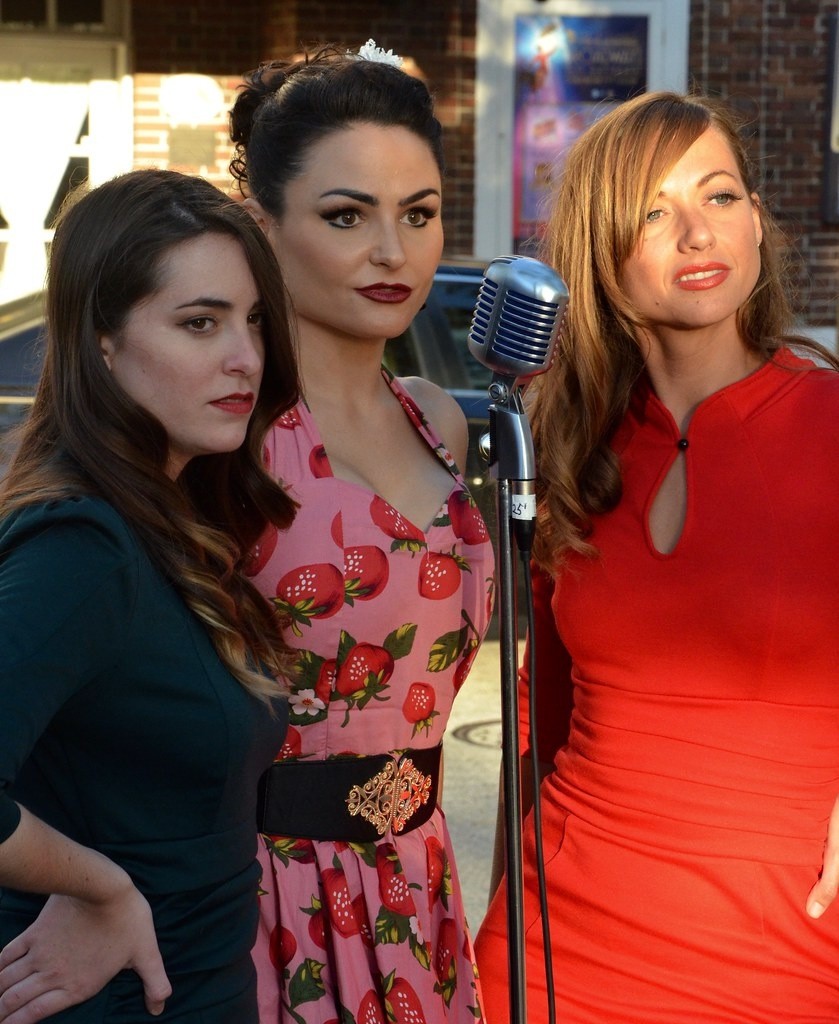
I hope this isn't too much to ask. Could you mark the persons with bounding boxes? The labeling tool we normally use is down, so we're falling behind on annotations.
[474,91,839,1021]
[227,57,498,1024]
[0,168,303,1024]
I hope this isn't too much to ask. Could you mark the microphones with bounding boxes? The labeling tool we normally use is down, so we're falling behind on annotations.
[468,256,570,547]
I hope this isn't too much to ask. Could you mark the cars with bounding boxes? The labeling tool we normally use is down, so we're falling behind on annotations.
[0,250,542,644]
